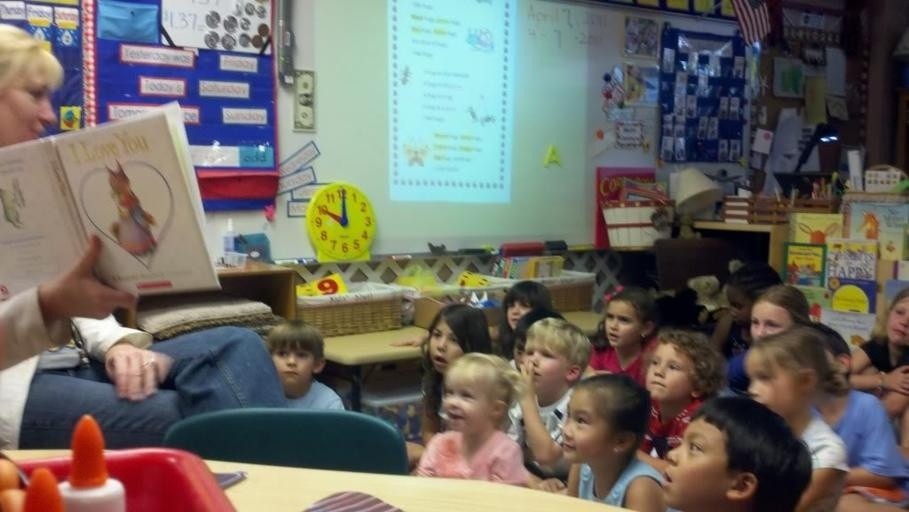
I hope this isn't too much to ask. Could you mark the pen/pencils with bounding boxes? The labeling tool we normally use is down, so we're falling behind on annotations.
[811,171,839,200]
[789,188,800,206]
[774,184,781,203]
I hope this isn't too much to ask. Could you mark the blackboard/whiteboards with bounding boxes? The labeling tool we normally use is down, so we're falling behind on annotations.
[94,0,749,268]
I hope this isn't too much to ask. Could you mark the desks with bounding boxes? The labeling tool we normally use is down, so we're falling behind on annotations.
[118,260,296,326]
[0,449,636,512]
[486,309,604,339]
[692,219,790,277]
[323,324,428,412]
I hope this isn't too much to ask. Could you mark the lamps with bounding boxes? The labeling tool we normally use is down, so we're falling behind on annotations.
[675,167,732,216]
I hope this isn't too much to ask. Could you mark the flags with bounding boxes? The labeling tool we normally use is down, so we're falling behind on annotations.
[728,1,773,45]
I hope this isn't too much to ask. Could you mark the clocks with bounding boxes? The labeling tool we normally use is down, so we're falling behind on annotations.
[304,183,379,264]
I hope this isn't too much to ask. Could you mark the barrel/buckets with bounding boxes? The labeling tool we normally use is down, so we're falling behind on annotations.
[597,198,675,248]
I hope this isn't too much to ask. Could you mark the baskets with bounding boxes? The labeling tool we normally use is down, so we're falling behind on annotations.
[296,270,595,339]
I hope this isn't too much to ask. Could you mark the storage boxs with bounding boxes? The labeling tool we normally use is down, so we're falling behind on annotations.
[360,388,425,440]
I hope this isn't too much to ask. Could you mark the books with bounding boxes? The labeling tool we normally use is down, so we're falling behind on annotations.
[0,103,223,296]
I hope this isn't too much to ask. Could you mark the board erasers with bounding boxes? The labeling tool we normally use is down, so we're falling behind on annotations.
[500,243,546,258]
[546,241,567,251]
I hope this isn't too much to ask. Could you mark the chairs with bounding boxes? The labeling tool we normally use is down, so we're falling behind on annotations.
[166,408,409,473]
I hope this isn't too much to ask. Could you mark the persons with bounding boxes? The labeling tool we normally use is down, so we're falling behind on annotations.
[410,280,592,491]
[0,25,284,456]
[541,264,909,510]
[267,321,350,414]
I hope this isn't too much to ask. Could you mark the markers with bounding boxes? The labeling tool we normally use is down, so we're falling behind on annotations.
[274,259,316,264]
[458,249,484,254]
[392,255,413,260]
